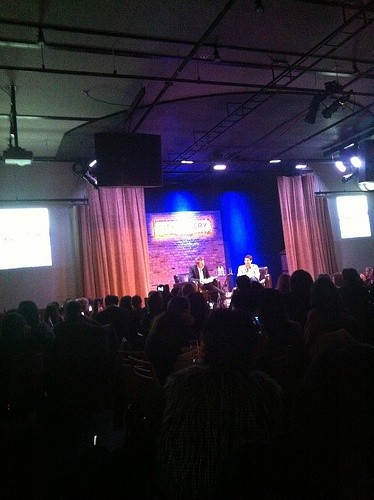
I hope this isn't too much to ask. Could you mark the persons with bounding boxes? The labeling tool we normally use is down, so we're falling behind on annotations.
[231,255,261,296]
[188,256,226,309]
[0,268,374,500]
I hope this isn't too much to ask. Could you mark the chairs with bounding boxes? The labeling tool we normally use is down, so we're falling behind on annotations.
[259,267,272,288]
[173,274,220,301]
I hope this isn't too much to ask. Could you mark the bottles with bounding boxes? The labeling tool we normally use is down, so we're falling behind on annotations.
[218,265,224,275]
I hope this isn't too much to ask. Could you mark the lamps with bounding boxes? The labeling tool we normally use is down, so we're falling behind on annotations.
[352,61,360,76]
[335,142,362,183]
[72,161,99,190]
[213,48,221,62]
[322,94,350,119]
[255,0,265,16]
[303,95,327,124]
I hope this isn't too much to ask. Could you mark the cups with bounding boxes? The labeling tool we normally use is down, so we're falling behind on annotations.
[229,268,232,274]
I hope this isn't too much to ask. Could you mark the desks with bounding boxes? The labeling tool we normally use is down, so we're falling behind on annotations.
[214,274,235,299]
